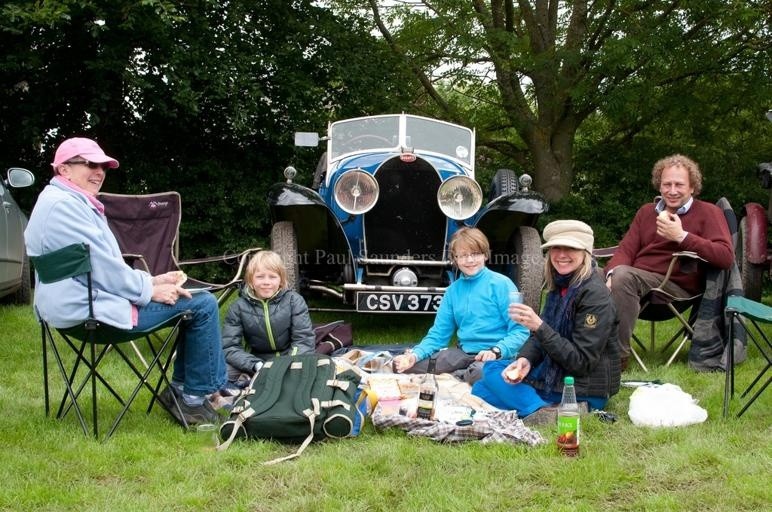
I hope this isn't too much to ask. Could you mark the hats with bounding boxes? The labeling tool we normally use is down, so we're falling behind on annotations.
[51,137,119,175]
[541,220,594,254]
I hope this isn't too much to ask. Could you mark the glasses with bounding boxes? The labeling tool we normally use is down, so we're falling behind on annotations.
[455,251,485,261]
[65,159,99,169]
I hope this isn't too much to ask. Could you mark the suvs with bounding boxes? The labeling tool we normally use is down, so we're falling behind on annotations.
[735,161,771,302]
[268,115,549,315]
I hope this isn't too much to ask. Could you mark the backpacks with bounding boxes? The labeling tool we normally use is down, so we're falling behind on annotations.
[220,353,361,444]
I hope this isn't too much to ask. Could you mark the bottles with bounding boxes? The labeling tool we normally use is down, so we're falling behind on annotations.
[556,376,581,456]
[417,355,438,419]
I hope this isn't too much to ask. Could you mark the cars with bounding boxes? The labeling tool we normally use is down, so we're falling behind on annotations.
[0,167,33,304]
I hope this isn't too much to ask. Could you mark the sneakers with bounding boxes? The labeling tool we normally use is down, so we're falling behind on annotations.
[157,384,183,408]
[170,396,226,425]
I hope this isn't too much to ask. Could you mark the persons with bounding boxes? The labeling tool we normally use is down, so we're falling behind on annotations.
[22,135,230,429]
[596,152,736,373]
[222,248,317,386]
[390,225,531,387]
[468,217,623,421]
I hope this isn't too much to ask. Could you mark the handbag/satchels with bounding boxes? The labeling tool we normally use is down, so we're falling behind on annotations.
[351,386,378,437]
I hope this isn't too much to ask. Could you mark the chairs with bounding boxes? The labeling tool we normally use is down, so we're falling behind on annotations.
[92,190,263,372]
[582,195,747,377]
[27,242,194,446]
[724,294,772,419]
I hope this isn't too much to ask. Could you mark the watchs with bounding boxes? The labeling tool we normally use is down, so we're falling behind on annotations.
[491,346,502,359]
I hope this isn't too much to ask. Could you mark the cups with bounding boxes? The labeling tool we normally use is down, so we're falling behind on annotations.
[509,291,524,305]
[197,424,218,448]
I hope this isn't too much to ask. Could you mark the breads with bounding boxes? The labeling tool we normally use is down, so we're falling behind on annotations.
[507,368,521,383]
[660,209,671,222]
[168,269,186,286]
